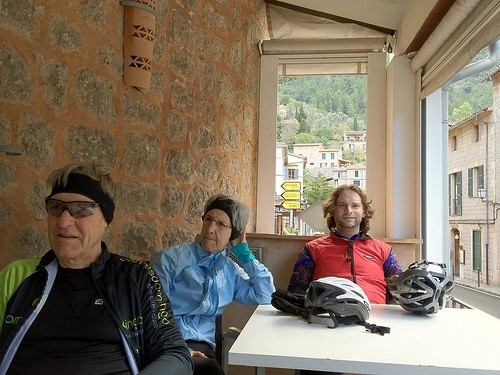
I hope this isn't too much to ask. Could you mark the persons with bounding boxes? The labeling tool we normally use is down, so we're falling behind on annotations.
[287,183,403,305]
[0,161,193,375]
[150,194,276,375]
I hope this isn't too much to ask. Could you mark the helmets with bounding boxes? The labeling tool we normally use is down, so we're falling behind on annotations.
[385,267,454,314]
[303,276,369,329]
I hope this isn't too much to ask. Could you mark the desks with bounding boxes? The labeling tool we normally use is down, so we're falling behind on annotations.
[228,304,500,375]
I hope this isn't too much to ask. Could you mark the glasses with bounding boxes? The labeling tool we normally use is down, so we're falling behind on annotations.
[201,213,233,230]
[45,197,99,219]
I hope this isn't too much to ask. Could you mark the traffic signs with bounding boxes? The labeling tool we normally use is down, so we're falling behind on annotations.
[281,181,301,209]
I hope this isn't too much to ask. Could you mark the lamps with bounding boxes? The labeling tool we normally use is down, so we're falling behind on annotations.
[120,0,158,92]
[478,186,491,203]
